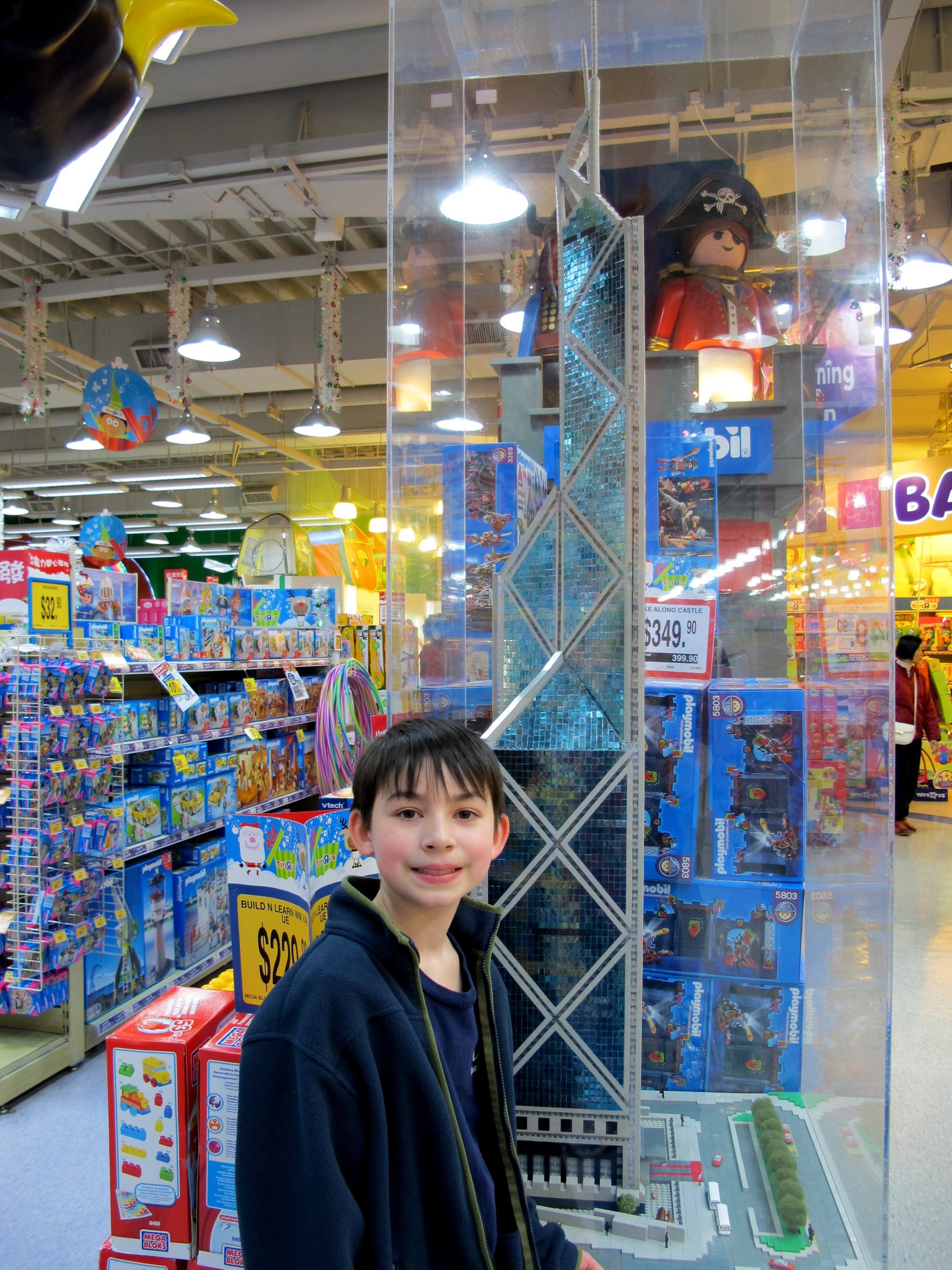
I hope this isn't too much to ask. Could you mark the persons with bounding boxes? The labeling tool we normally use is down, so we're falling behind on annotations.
[646,169,774,402]
[893,636,943,835]
[232,715,607,1270]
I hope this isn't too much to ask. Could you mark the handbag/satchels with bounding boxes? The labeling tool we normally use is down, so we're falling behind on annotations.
[882,721,915,746]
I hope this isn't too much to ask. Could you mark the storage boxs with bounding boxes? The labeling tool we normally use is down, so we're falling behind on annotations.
[98,677,806,1270]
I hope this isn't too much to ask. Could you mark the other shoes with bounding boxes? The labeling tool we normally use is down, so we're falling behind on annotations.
[902,818,916,832]
[893,820,911,836]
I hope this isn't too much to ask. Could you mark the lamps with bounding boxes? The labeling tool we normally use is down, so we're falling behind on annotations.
[1,29,952,580]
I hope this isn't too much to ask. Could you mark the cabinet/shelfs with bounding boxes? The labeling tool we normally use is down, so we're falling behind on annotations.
[0,659,330,1106]
